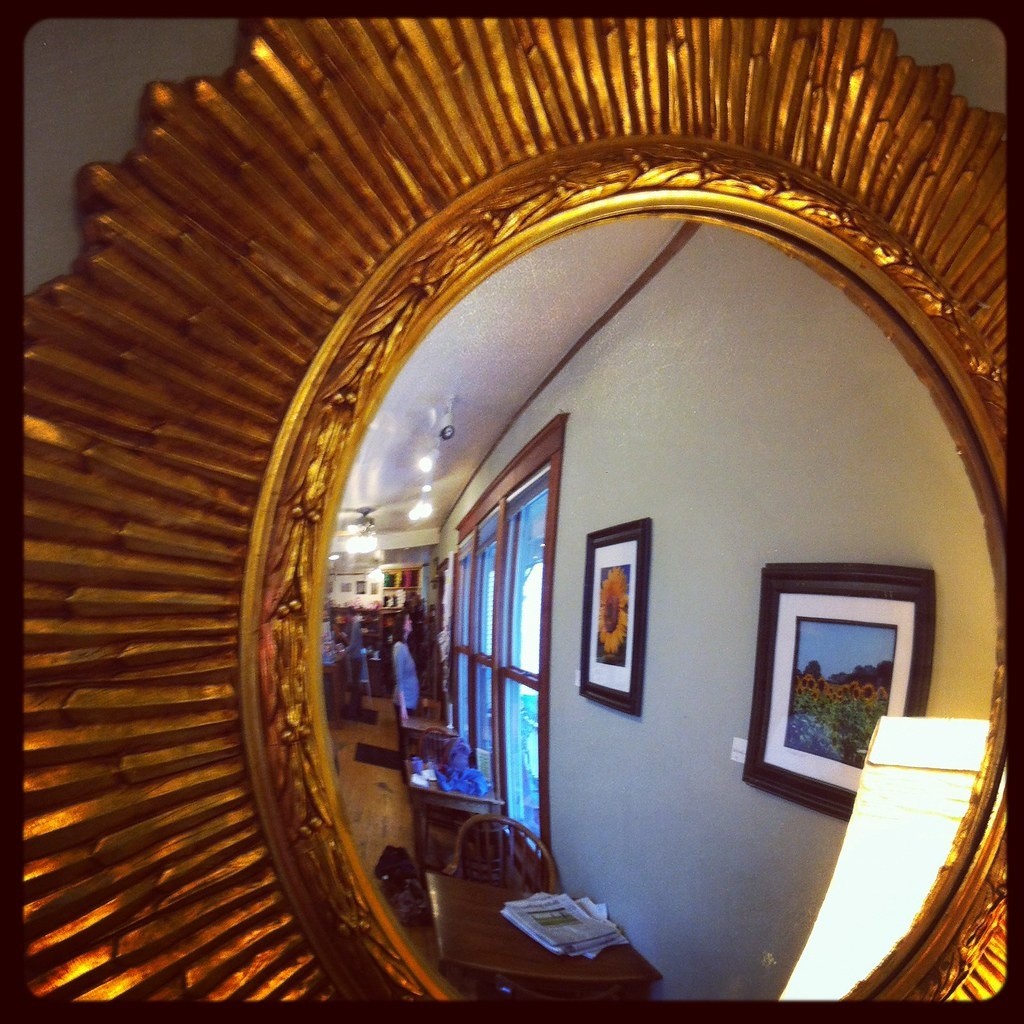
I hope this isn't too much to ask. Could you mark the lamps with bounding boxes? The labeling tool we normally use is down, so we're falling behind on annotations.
[778,716,990,1001]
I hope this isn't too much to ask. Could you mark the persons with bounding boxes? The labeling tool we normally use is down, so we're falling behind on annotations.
[330,624,349,647]
[390,610,420,786]
[336,606,362,714]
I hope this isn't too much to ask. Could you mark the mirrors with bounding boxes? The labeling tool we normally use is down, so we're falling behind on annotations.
[19,17,1006,1000]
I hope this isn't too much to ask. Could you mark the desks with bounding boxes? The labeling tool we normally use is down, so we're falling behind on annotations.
[325,664,346,721]
[404,760,505,885]
[400,714,459,759]
[425,871,663,1000]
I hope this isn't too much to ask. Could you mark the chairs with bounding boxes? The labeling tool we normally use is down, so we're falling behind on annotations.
[443,814,558,894]
[418,725,450,763]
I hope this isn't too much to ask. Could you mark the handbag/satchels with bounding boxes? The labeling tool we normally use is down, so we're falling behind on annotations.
[349,658,362,676]
[374,845,431,928]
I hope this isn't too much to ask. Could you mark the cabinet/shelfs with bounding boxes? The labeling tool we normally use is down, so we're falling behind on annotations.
[330,606,387,697]
[381,567,421,613]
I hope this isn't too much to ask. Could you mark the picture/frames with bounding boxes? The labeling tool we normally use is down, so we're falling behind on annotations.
[742,561,938,822]
[579,517,652,718]
